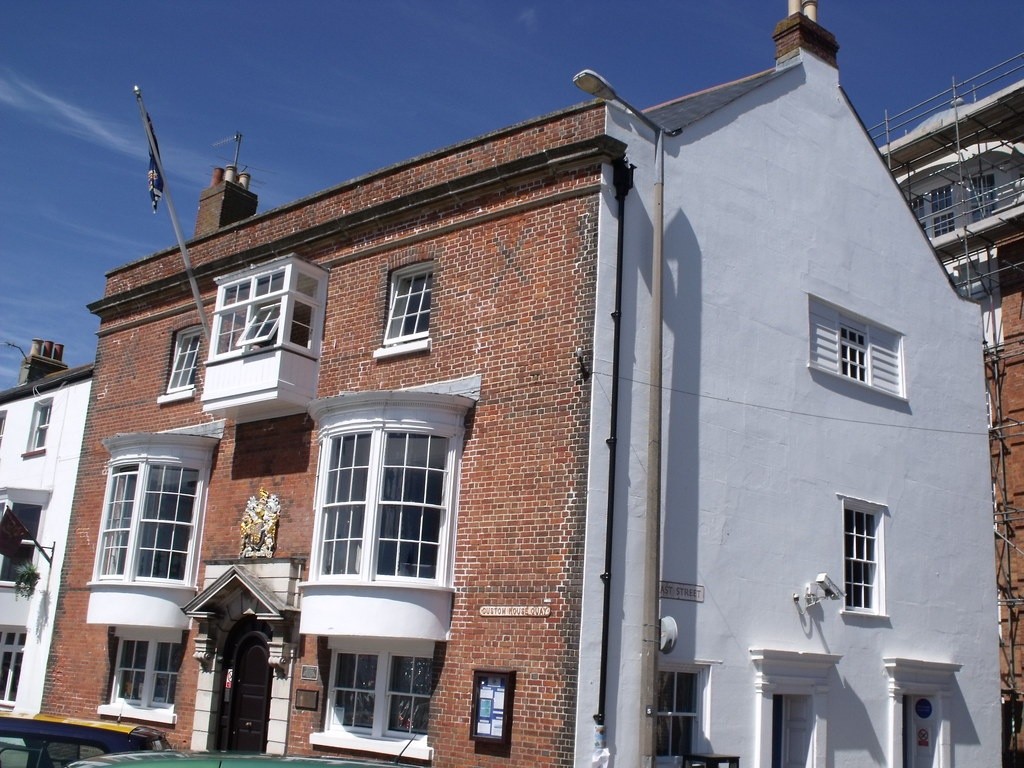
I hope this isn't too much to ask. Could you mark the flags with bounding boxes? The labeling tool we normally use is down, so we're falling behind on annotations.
[0,509,27,559]
[145,112,164,213]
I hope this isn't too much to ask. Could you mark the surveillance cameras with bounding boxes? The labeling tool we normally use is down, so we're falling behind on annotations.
[816,573,847,600]
